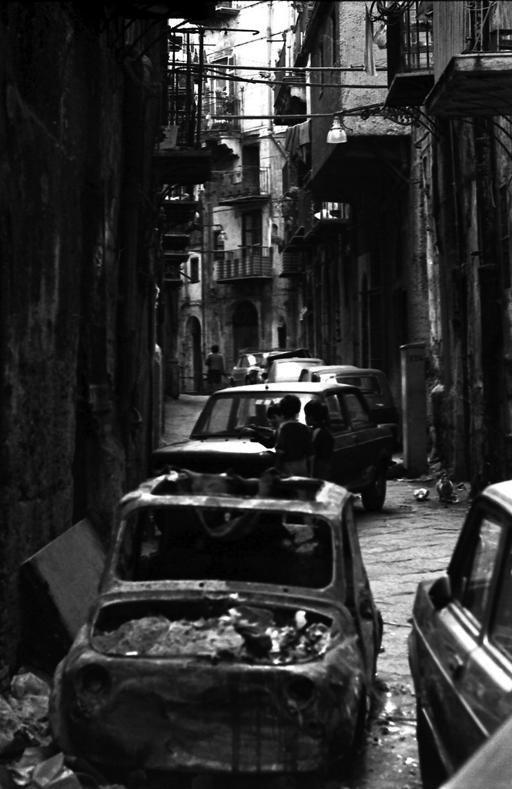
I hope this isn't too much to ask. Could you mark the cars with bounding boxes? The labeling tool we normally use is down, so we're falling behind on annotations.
[226,349,397,429]
[53,469,384,787]
[405,477,510,786]
[144,381,398,512]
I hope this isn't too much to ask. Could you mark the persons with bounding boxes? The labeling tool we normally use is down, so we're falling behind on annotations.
[304,399,335,479]
[266,404,286,428]
[205,345,226,393]
[240,395,312,477]
[427,368,458,474]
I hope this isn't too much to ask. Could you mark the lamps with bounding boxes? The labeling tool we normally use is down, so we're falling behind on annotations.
[325,113,347,144]
